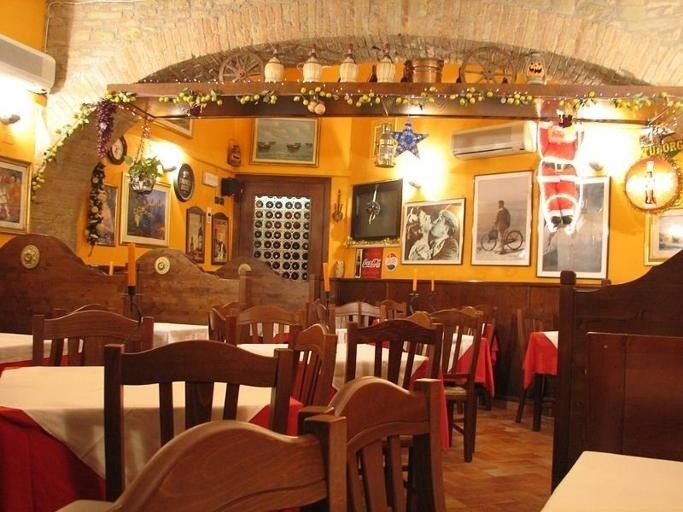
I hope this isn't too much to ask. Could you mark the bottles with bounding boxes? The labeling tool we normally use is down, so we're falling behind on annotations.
[355,250,362,276]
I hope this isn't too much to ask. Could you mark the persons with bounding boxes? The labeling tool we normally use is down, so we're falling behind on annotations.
[429,209,459,261]
[7,173,21,222]
[133,196,166,238]
[543,192,603,273]
[405,206,444,260]
[494,199,511,253]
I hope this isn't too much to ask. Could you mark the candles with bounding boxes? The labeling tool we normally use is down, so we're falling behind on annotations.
[128,242,137,286]
[431,268,435,292]
[323,261,329,292]
[412,269,418,291]
[109,261,114,276]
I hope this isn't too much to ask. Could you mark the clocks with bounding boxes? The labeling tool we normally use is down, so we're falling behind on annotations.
[108,135,128,164]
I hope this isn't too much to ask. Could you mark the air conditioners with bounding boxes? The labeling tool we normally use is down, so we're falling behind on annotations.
[451,121,537,158]
[1,35,57,95]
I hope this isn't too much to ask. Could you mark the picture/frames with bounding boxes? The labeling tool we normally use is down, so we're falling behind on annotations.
[211,212,229,263]
[645,210,683,266]
[0,155,33,235]
[400,198,465,265]
[186,206,207,266]
[471,171,534,268]
[99,183,117,248]
[159,119,195,139]
[536,175,608,279]
[121,173,172,247]
[250,118,321,166]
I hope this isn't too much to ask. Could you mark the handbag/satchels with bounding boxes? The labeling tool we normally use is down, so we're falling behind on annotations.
[488,230,498,239]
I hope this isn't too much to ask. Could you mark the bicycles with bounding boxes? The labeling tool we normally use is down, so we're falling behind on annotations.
[481,225,523,251]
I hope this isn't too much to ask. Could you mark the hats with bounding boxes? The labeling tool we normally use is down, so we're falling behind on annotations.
[441,210,457,227]
